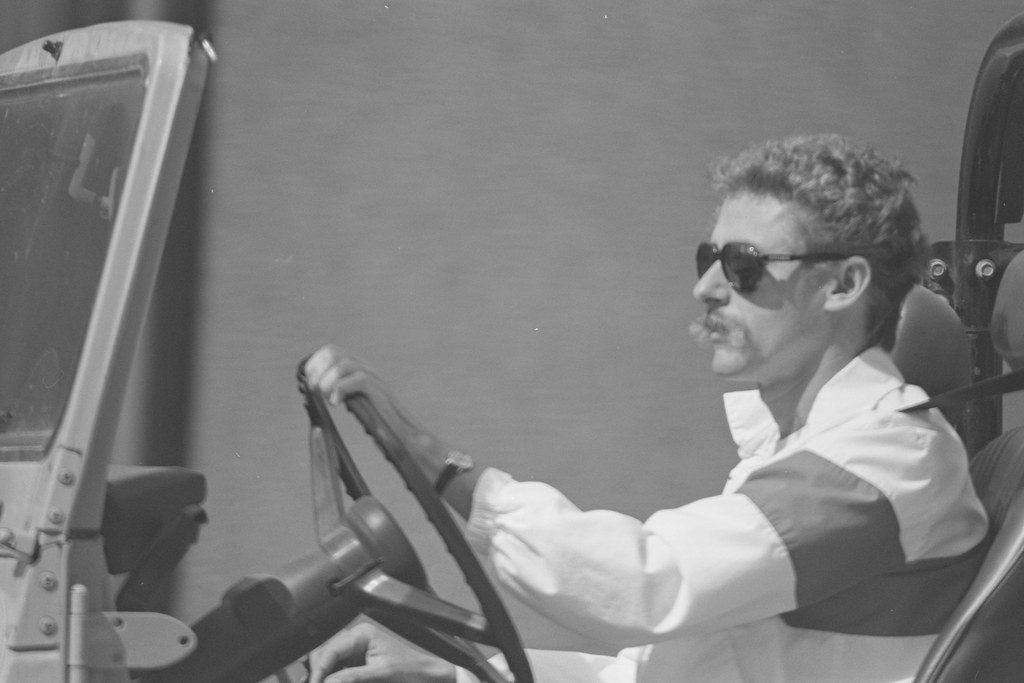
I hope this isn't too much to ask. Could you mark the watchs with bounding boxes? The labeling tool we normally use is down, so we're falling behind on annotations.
[434,450,474,497]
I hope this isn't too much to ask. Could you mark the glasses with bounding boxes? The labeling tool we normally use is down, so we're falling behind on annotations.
[695,240,871,294]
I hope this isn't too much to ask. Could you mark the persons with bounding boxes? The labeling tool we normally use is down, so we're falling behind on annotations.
[303,133,991,683]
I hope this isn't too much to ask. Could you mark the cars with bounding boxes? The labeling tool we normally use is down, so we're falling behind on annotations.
[0,11,1024,683]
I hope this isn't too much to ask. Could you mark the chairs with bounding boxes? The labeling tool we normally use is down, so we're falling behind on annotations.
[878,248,1024,683]
